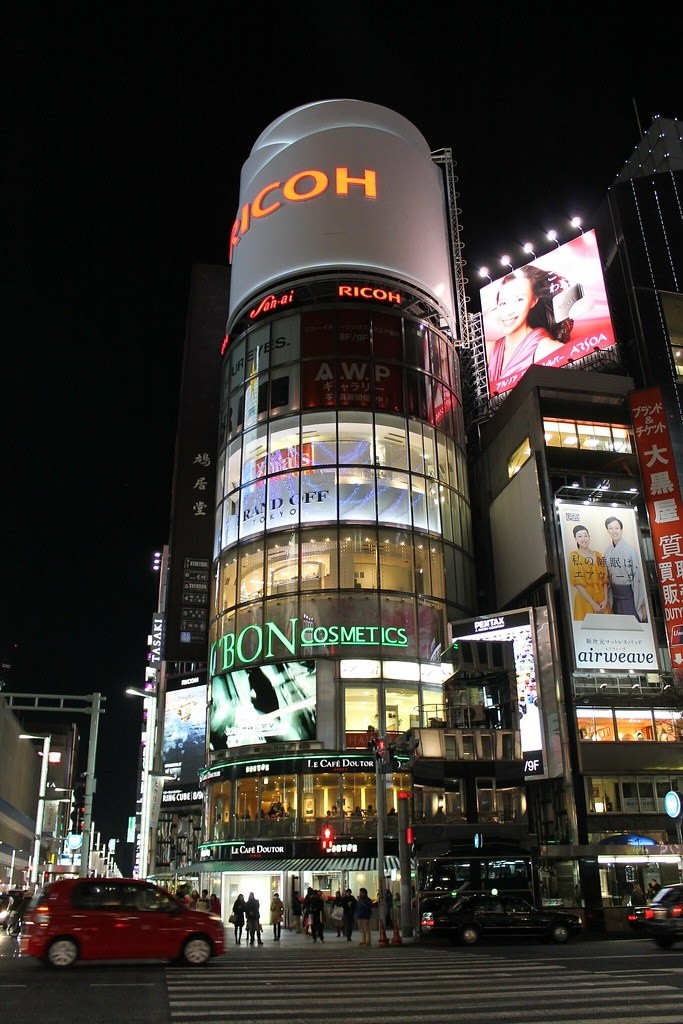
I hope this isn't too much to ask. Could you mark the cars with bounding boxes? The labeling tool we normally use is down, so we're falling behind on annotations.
[626,883,683,949]
[0,889,29,936]
[17,878,225,969]
[419,893,583,947]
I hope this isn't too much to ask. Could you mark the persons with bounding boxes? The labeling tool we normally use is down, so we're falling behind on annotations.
[6,882,26,959]
[357,888,373,945]
[216,802,297,822]
[435,806,444,816]
[487,263,567,382]
[630,878,662,906]
[232,892,263,946]
[270,893,284,941]
[332,887,356,944]
[603,517,648,624]
[327,805,396,819]
[386,888,400,931]
[568,525,611,621]
[184,889,221,916]
[291,887,324,943]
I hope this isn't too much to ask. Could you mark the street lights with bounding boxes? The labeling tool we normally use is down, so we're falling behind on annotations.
[17,735,54,897]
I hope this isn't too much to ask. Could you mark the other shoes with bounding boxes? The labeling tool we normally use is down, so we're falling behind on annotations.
[360,942,371,947]
[273,937,280,941]
[235,941,241,945]
[258,942,264,945]
[250,941,254,945]
[336,934,341,937]
[312,939,317,943]
[319,939,325,943]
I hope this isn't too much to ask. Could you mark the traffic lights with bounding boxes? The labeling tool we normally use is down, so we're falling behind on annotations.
[366,735,388,751]
[373,750,390,765]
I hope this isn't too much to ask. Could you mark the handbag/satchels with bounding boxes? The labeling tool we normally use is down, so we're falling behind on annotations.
[228,911,238,924]
[331,905,344,921]
